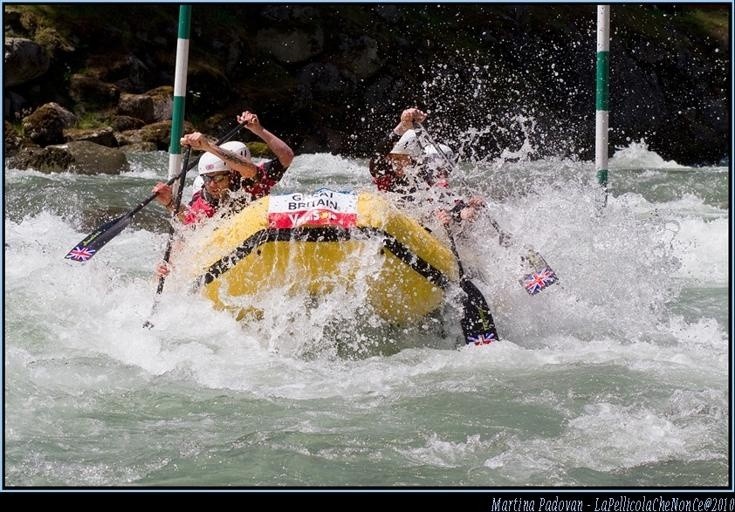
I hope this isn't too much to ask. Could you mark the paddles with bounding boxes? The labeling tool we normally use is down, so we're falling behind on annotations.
[65,112,258,262]
[444,223,499,346]
[418,122,559,296]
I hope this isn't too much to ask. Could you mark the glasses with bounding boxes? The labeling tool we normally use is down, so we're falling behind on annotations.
[201,172,233,183]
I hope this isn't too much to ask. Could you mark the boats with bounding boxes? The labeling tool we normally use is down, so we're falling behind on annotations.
[189,189,463,331]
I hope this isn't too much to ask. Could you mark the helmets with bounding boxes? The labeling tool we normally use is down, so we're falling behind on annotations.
[219,140,253,162]
[196,150,231,176]
[424,144,455,167]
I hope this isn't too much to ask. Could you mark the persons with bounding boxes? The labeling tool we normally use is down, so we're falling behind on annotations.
[412,135,486,250]
[149,109,295,277]
[151,127,269,232]
[368,106,486,251]
